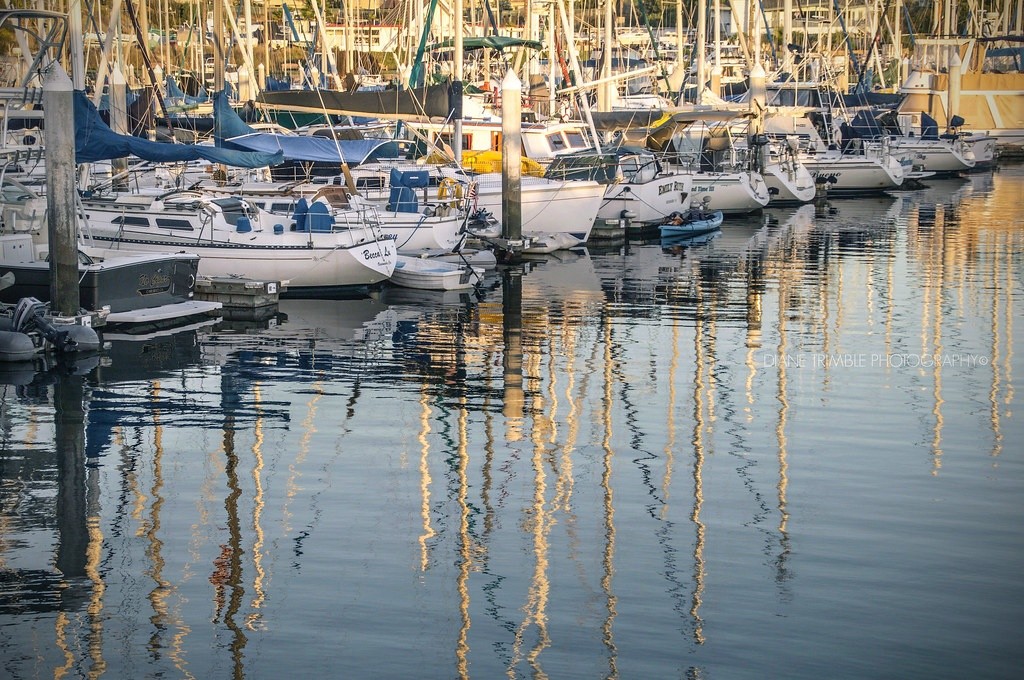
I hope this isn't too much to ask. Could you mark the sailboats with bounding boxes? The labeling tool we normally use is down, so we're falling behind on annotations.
[1,0,1024,365]
